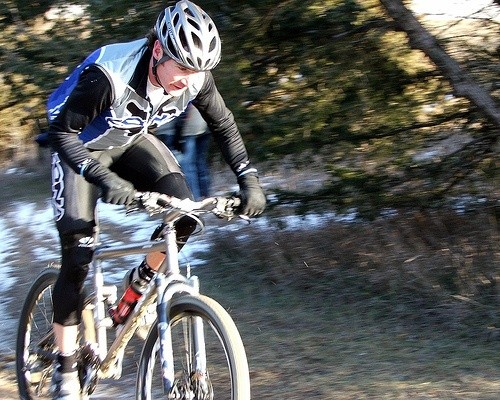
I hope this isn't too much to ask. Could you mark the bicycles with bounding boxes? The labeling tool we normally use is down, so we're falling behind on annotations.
[15,191,280,400]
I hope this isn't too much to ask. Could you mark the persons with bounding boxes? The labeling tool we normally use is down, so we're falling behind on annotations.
[46,0,268,400]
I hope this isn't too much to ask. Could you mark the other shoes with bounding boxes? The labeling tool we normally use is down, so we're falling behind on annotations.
[131,267,150,294]
[49,366,81,400]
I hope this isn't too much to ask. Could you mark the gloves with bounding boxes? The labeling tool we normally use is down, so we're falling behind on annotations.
[237,172,266,215]
[85,164,134,206]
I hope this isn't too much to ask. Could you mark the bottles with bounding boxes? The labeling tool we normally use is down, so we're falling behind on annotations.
[114,279,148,323]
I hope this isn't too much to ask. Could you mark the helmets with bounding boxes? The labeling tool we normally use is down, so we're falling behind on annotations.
[154,0,222,71]
